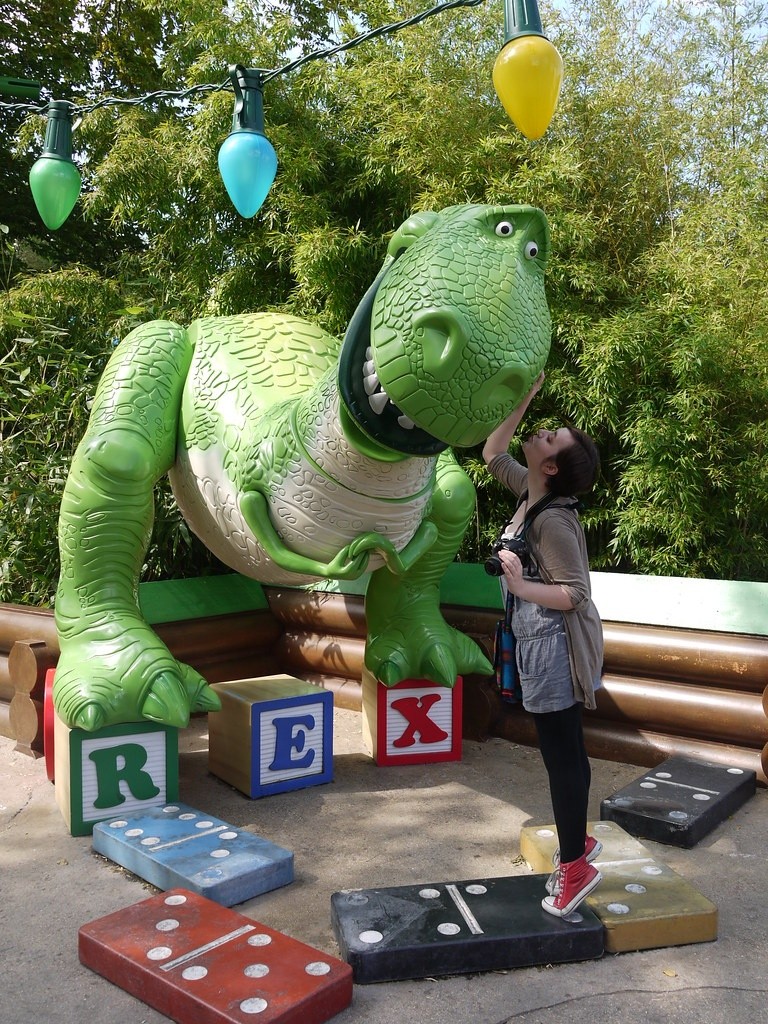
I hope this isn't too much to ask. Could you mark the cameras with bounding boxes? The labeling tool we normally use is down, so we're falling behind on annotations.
[484,537,529,577]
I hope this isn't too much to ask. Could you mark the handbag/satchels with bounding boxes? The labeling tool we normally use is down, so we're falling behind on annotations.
[492,618,521,704]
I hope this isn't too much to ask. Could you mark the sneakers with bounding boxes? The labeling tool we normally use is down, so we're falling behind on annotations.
[545,833,603,896]
[542,854,602,917]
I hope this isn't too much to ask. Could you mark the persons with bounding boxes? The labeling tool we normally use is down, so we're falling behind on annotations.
[482,372,603,916]
